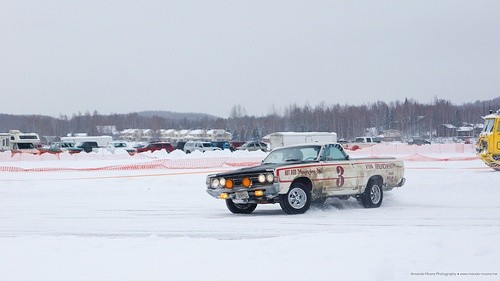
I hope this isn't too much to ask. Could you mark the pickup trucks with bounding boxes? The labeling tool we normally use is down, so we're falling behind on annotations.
[342,136,385,151]
[204,142,407,215]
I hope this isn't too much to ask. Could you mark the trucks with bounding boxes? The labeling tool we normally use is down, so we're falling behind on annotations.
[0,129,43,154]
[59,133,113,153]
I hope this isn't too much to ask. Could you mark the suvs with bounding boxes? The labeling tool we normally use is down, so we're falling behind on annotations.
[135,141,175,154]
[176,140,223,155]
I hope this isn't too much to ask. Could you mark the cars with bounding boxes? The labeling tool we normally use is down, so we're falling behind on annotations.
[213,139,268,153]
[107,141,136,155]
[408,137,431,146]
